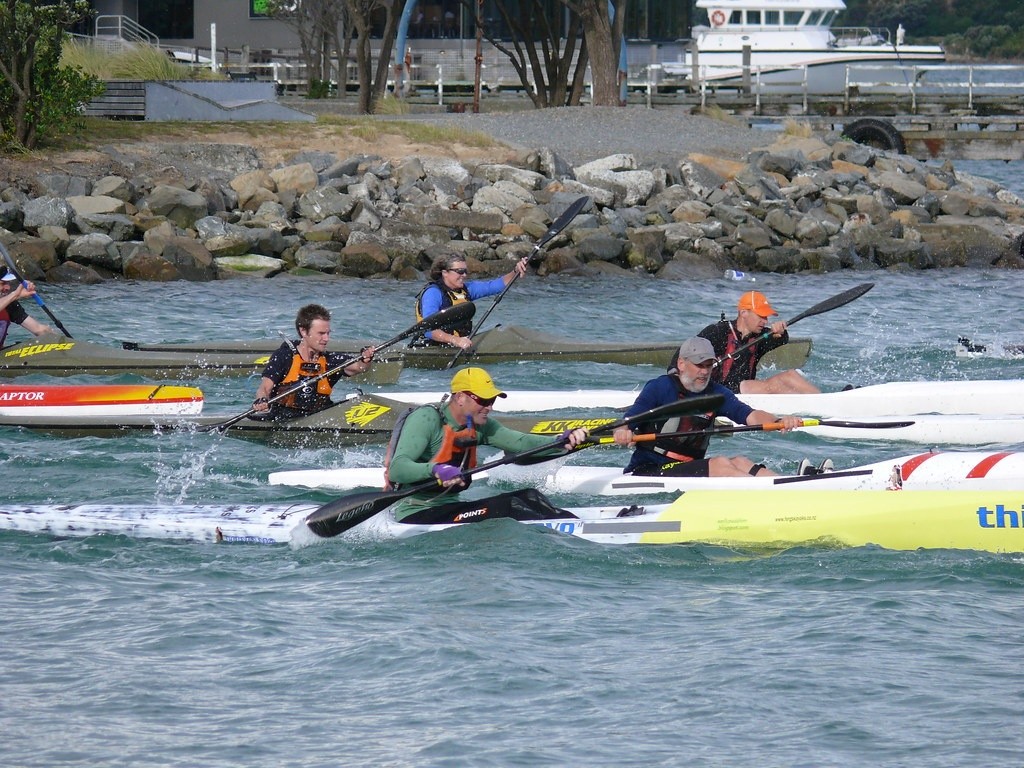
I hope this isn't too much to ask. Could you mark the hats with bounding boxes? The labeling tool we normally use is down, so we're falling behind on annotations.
[679,336,720,365]
[0,265,17,281]
[451,367,507,399]
[738,290,779,318]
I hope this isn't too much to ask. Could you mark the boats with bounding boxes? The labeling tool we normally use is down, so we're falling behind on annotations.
[219,381,1024,446]
[267,451,1024,501]
[0,383,250,441]
[0,488,1024,553]
[0,326,814,374]
[623,0,945,96]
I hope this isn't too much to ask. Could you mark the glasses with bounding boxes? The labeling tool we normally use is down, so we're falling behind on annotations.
[463,390,496,407]
[447,268,468,275]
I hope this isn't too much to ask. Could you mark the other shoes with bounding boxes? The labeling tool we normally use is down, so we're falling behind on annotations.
[617,505,638,518]
[842,384,853,392]
[797,457,815,475]
[817,458,834,473]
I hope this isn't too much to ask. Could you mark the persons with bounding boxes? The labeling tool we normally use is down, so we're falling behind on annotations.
[253,304,375,420]
[383,367,647,525]
[612,338,834,477]
[667,291,862,394]
[416,252,528,350]
[0,266,55,346]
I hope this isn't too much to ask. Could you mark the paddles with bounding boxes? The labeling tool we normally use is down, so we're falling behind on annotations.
[179,301,476,435]
[709,283,875,368]
[441,195,590,372]
[580,418,915,444]
[0,242,73,339]
[304,394,726,537]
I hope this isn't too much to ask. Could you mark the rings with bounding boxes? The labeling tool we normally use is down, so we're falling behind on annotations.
[464,344,466,346]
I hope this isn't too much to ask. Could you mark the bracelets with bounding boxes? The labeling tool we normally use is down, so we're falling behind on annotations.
[775,418,782,431]
[514,269,516,273]
